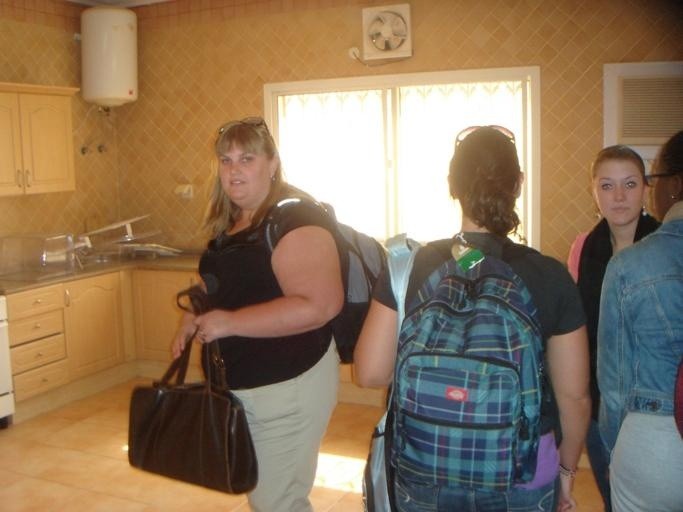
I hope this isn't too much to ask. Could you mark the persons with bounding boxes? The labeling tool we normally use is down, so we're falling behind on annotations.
[574,144,664,512]
[595,130,683,512]
[170,115,346,512]
[352,127,594,511]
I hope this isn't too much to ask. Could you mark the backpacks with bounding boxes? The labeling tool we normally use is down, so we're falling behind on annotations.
[390,239,545,495]
[265,194,384,365]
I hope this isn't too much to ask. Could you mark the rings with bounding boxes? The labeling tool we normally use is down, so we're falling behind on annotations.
[199,328,205,335]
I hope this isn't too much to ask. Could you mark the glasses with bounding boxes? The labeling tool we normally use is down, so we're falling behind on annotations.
[216,117,275,148]
[644,174,671,188]
[455,125,515,148]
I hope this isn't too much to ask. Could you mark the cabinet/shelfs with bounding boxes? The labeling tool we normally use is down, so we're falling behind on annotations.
[1,83,78,196]
[131,269,197,369]
[68,272,123,385]
[6,284,71,404]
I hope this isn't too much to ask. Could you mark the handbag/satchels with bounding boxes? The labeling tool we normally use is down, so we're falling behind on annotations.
[361,409,396,512]
[128,380,259,494]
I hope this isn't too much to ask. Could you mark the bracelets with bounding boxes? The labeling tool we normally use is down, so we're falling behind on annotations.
[558,465,577,478]
